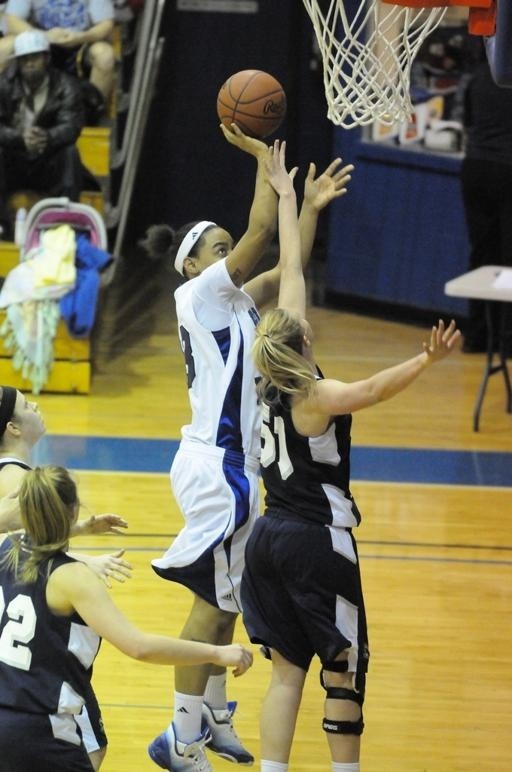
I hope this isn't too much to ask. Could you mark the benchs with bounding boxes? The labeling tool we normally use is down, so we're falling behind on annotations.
[0,24,125,393]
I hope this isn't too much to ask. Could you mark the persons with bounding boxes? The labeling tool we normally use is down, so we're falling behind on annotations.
[463,62,512,353]
[0,0,114,125]
[151,122,356,772]
[239,138,462,772]
[1,30,86,243]
[2,465,253,772]
[0,385,133,589]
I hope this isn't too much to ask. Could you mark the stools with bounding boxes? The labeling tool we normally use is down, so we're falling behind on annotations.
[443,265,512,430]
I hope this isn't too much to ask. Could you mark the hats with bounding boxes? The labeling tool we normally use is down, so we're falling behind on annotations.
[8,30,50,59]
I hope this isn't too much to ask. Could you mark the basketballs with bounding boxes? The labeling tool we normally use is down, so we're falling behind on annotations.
[217,71,286,140]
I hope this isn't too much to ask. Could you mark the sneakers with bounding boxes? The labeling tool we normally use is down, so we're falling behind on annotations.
[148,722,214,771]
[202,702,256,765]
